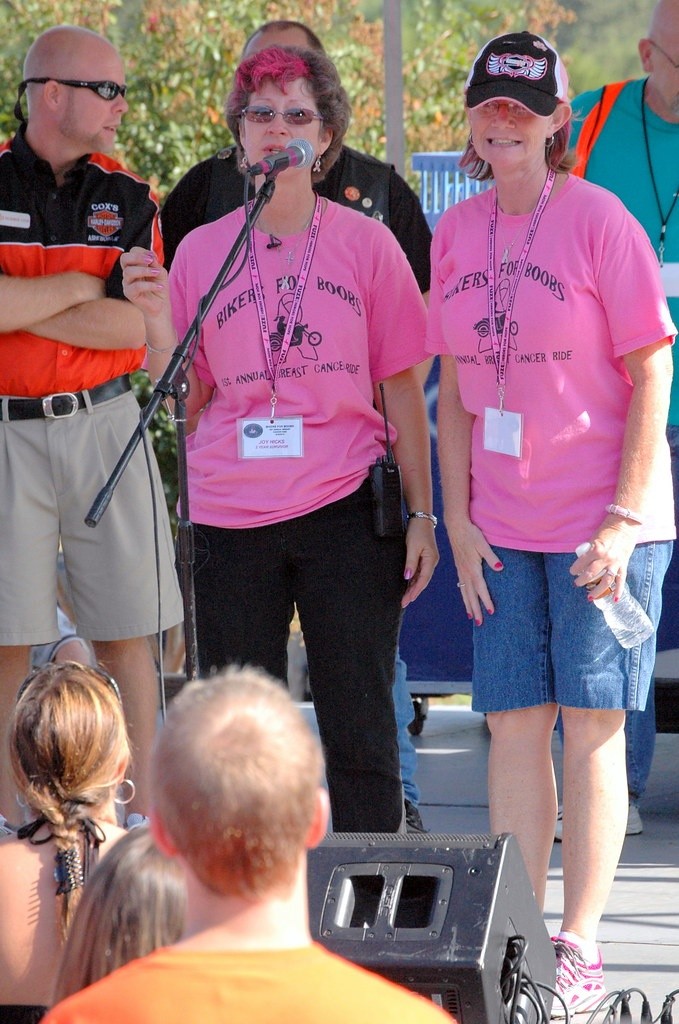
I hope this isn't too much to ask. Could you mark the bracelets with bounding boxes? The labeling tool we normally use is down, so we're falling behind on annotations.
[146,331,178,353]
[406,512,437,530]
[605,504,644,524]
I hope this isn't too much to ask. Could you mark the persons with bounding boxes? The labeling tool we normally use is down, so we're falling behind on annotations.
[426,30,677,1018]
[159,21,433,832]
[0,594,456,1024]
[555,0,679,843]
[119,45,437,833]
[0,27,187,833]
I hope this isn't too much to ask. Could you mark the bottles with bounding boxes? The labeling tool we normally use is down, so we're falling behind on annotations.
[575,542,655,650]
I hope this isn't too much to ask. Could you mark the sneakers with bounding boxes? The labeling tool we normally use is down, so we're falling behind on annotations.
[551,795,644,841]
[550,941,606,1016]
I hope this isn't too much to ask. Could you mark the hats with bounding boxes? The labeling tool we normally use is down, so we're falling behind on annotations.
[462,30,568,117]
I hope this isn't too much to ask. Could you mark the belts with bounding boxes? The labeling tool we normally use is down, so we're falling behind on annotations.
[0,374,133,422]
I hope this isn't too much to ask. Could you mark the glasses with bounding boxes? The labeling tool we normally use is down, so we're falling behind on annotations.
[240,106,326,126]
[43,76,128,99]
[480,99,531,117]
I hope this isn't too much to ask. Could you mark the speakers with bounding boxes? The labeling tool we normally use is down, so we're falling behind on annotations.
[306,832,555,1024]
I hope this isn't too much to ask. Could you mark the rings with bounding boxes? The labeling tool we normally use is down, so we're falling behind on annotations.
[606,570,618,577]
[457,583,465,587]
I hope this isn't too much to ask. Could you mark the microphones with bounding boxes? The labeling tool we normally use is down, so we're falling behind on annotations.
[247,138,314,176]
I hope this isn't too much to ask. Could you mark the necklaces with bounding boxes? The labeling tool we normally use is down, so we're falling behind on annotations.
[497,205,538,263]
[256,213,315,288]
